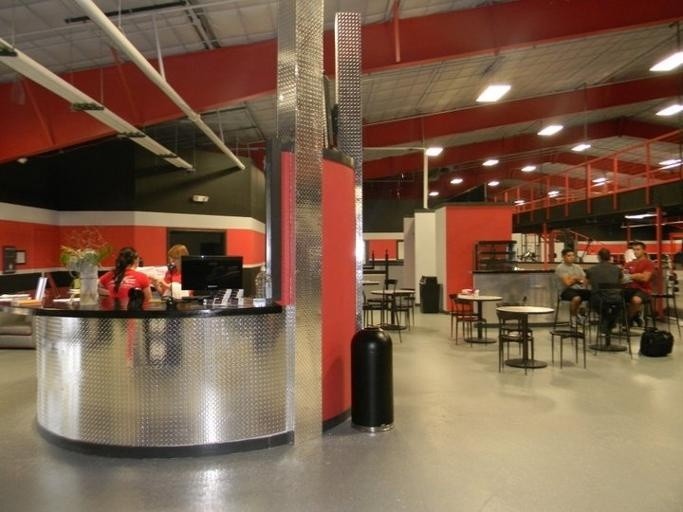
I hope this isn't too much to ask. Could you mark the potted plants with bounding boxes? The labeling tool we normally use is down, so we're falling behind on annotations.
[60,228,114,305]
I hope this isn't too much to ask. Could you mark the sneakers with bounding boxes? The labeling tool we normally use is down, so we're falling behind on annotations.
[571,314,589,326]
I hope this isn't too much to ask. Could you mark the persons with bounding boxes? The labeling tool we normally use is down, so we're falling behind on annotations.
[148,245,193,298]
[585,248,624,333]
[552,248,592,329]
[96,246,153,300]
[622,242,656,327]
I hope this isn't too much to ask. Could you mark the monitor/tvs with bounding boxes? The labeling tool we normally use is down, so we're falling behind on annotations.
[181,252,244,296]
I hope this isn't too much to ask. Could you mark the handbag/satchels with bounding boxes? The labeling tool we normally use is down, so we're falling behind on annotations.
[641,326,673,356]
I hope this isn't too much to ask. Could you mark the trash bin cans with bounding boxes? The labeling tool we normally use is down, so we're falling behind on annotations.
[352,327,393,433]
[419,276,439,313]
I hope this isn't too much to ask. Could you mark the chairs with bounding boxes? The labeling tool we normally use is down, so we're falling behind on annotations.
[365,278,415,344]
[448,266,683,376]
[0,276,47,348]
